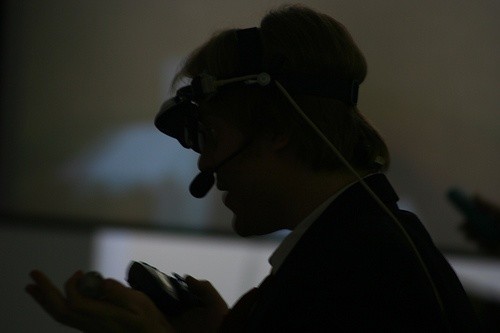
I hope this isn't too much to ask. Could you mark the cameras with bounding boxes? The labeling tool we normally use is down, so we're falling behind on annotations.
[127,262,211,319]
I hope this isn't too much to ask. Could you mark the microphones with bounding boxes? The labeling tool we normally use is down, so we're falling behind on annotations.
[189,128,262,198]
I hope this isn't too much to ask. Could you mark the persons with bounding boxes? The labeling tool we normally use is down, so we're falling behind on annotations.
[25,3,477,333]
